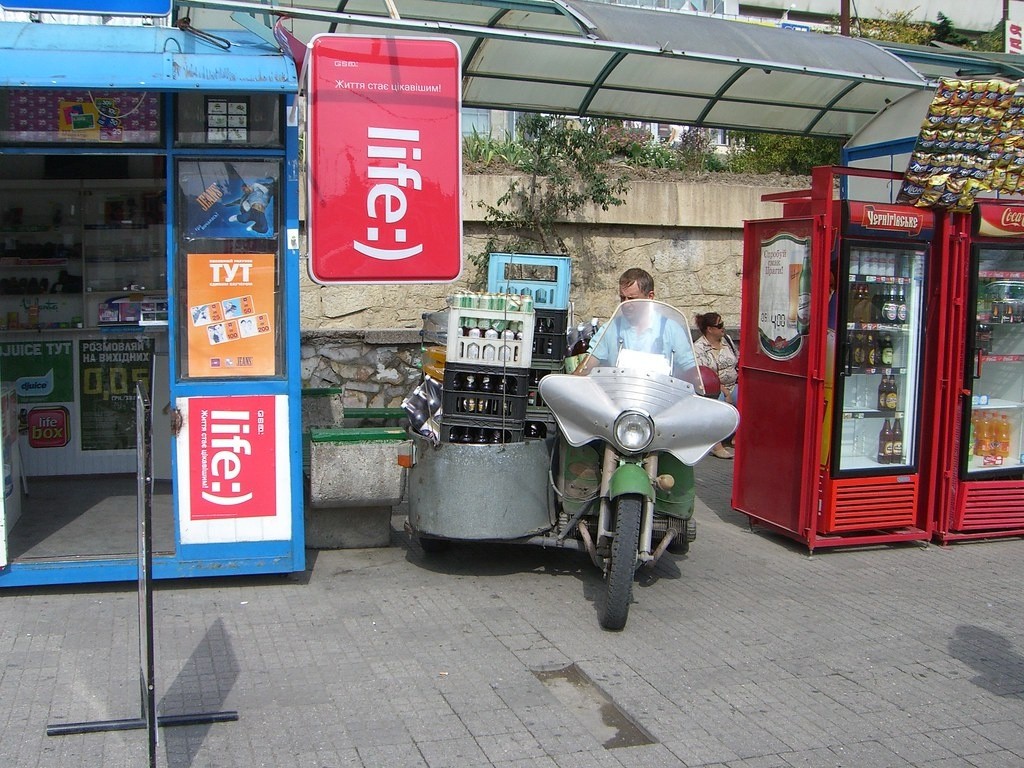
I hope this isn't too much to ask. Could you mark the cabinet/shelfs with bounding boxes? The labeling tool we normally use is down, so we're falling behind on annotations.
[85,178,169,331]
[0,178,85,333]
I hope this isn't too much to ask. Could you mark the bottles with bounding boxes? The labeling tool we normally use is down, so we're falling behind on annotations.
[847,282,907,324]
[797,240,812,334]
[850,332,893,367]
[976,278,1024,356]
[877,374,897,411]
[879,418,901,465]
[968,410,1008,461]
[399,308,599,443]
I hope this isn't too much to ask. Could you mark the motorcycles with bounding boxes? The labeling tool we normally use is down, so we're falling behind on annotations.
[408,298,740,635]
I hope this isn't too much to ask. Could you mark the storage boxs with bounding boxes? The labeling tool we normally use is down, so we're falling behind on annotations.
[439,252,570,444]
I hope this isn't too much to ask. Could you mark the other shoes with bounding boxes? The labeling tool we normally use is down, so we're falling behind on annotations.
[709,448,734,459]
[726,433,737,446]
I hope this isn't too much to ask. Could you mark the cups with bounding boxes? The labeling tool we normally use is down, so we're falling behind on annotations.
[788,251,803,327]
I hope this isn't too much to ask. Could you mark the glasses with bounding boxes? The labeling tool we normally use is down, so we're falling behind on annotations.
[710,321,724,329]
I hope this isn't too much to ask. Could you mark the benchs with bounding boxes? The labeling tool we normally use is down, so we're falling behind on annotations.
[301,386,407,550]
[344,407,408,418]
[690,329,741,355]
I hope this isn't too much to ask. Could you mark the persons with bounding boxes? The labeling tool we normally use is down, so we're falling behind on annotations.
[828,273,836,331]
[240,320,256,336]
[191,307,206,326]
[693,312,739,458]
[572,268,696,376]
[854,284,881,324]
[224,176,274,234]
[208,324,227,344]
[224,302,237,318]
